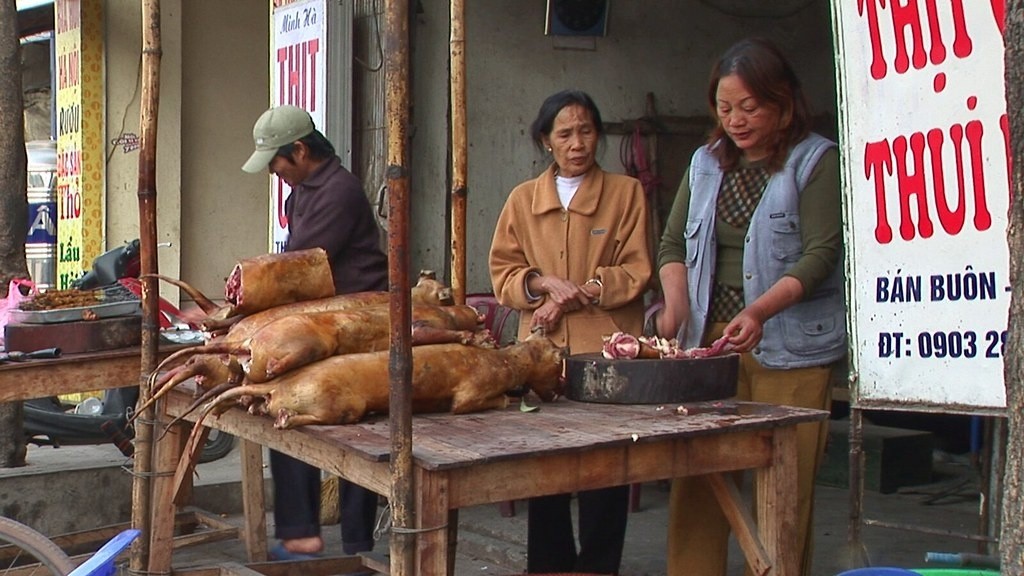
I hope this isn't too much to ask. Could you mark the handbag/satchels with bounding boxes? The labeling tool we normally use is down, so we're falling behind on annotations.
[0,278,39,345]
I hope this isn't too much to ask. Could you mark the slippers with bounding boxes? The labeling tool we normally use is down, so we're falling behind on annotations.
[270,539,328,559]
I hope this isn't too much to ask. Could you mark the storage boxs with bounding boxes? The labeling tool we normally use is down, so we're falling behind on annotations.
[817,421,933,494]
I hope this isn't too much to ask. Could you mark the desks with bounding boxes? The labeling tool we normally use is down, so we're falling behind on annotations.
[0,343,207,575]
[147,380,829,576]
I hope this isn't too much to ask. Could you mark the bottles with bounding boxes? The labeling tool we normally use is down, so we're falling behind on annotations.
[77,397,102,416]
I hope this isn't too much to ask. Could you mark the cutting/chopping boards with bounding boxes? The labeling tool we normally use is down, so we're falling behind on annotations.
[564,351,740,403]
[5,314,143,356]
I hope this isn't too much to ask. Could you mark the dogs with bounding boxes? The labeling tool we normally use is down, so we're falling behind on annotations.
[187,327,571,479]
[122,247,497,465]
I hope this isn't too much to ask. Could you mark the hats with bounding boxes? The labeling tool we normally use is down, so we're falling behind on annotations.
[242,105,314,172]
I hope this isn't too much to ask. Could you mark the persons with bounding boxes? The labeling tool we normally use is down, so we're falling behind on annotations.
[241,106,388,563]
[644,38,846,576]
[488,89,652,574]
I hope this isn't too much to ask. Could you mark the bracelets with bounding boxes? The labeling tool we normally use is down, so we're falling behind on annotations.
[584,279,602,305]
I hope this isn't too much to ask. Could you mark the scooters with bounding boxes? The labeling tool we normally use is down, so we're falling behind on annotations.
[0,238,234,467]
